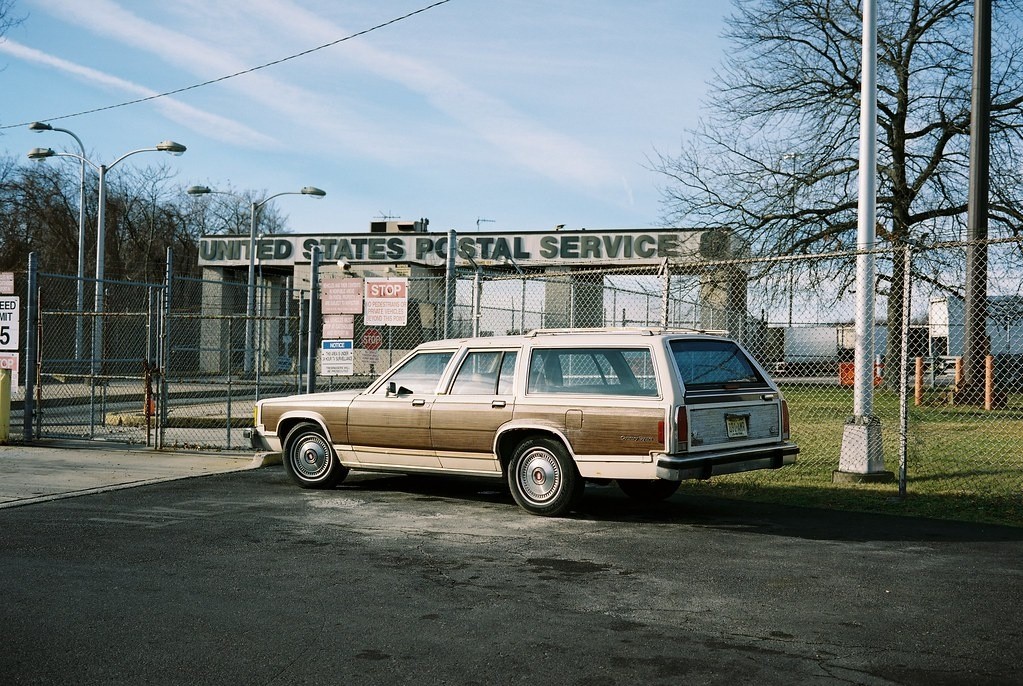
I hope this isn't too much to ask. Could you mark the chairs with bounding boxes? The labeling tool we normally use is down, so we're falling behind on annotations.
[457,372,497,394]
[528,372,546,391]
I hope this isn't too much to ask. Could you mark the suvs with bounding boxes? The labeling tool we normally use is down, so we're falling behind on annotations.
[256,326,802,520]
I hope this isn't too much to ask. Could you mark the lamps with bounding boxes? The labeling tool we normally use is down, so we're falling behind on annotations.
[337,257,355,278]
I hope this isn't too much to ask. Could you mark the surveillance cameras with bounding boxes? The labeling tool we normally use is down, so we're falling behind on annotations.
[337,260,352,270]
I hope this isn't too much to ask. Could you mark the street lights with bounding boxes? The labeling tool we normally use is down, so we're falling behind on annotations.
[26,140,187,389]
[185,184,328,397]
[28,121,86,362]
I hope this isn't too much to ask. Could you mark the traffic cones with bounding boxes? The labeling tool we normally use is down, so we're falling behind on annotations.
[136,386,160,416]
